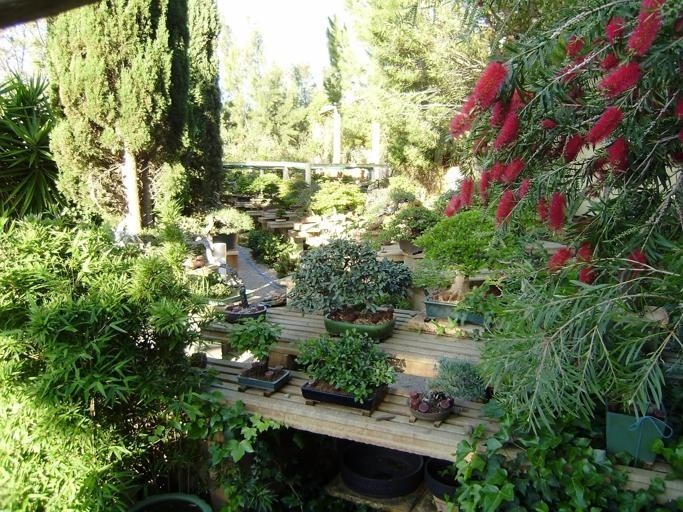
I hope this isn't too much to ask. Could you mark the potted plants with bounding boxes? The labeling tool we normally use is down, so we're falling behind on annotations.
[221,315,293,397]
[199,195,506,342]
[407,388,456,428]
[293,327,399,418]
